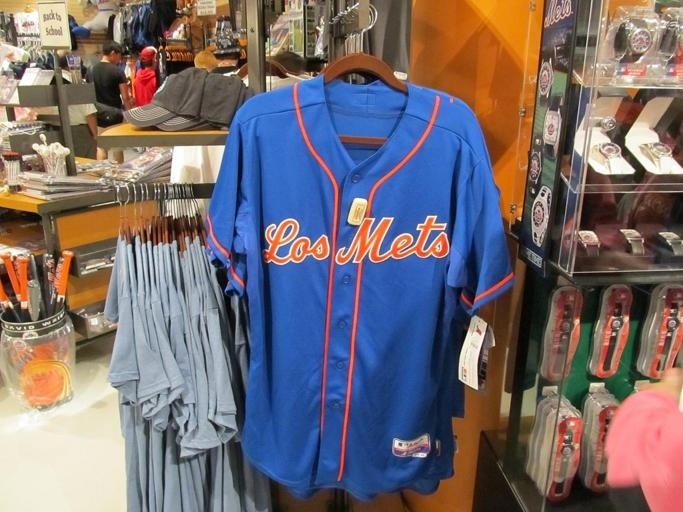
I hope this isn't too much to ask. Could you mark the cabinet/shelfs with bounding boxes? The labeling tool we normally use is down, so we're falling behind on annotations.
[0,155,161,359]
[504,1,681,512]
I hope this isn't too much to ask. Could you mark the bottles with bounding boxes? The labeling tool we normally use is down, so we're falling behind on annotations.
[1,306,77,411]
[215,14,235,50]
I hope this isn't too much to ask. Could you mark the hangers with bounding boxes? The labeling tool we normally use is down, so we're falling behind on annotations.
[110,181,209,254]
[319,4,408,148]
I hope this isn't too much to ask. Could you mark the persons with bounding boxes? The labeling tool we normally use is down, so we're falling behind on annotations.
[92,39,130,164]
[601,367,683,512]
[132,46,158,106]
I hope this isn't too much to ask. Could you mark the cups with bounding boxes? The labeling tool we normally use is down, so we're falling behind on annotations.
[67,54,82,85]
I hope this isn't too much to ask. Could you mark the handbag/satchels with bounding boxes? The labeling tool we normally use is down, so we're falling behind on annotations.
[95,102,123,127]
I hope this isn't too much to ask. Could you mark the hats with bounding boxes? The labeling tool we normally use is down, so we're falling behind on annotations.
[123,67,249,132]
[140,47,155,65]
